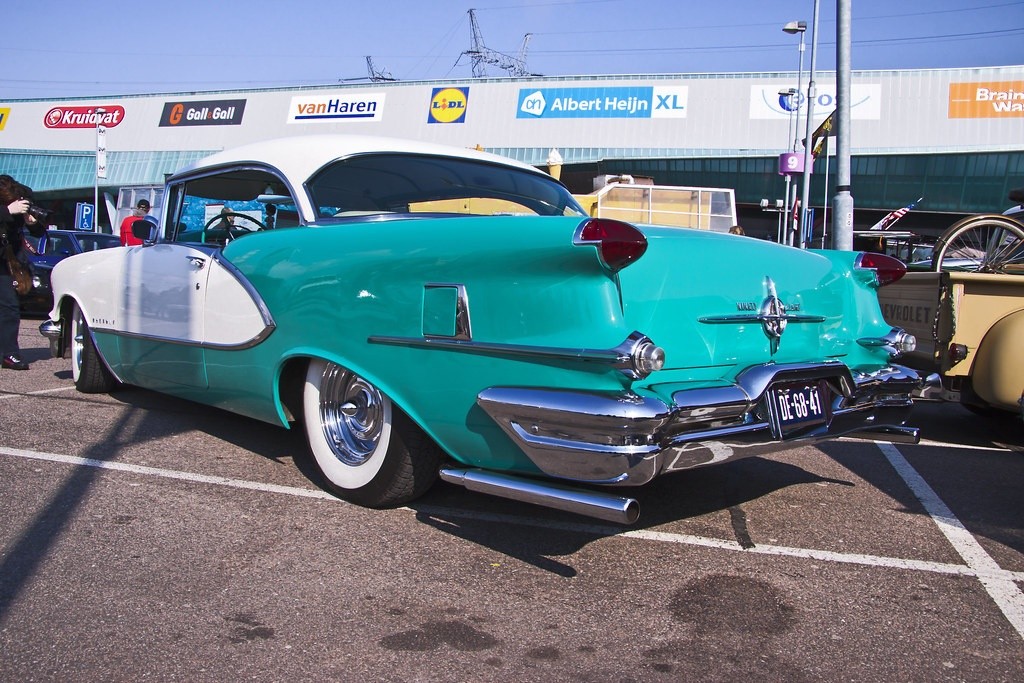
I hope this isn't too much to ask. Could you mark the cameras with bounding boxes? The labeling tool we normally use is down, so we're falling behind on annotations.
[19,197,54,223]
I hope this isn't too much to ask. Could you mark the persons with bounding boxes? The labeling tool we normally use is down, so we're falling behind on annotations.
[0,175,46,370]
[729,226,745,235]
[212,207,238,230]
[120,199,150,247]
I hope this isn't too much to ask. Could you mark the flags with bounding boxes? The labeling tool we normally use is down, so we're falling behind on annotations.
[802,109,837,159]
[792,197,798,230]
[870,201,918,230]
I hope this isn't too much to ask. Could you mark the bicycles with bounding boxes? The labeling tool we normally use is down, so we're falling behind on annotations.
[931,186,1024,274]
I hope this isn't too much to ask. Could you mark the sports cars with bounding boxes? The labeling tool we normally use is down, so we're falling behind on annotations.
[39,139,944,526]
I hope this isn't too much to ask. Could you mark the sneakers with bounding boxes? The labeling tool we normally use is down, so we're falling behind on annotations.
[2,354,29,370]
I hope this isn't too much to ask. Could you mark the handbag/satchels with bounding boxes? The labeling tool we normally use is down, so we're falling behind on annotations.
[8,250,33,298]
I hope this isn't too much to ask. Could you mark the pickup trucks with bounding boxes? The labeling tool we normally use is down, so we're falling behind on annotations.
[879,268,1024,414]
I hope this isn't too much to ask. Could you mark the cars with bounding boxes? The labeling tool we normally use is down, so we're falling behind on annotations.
[21,230,125,317]
[886,241,986,270]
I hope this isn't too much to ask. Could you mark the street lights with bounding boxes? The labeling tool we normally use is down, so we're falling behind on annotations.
[781,19,807,247]
[779,88,796,247]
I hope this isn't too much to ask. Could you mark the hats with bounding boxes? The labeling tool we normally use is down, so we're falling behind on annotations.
[137,199,149,209]
